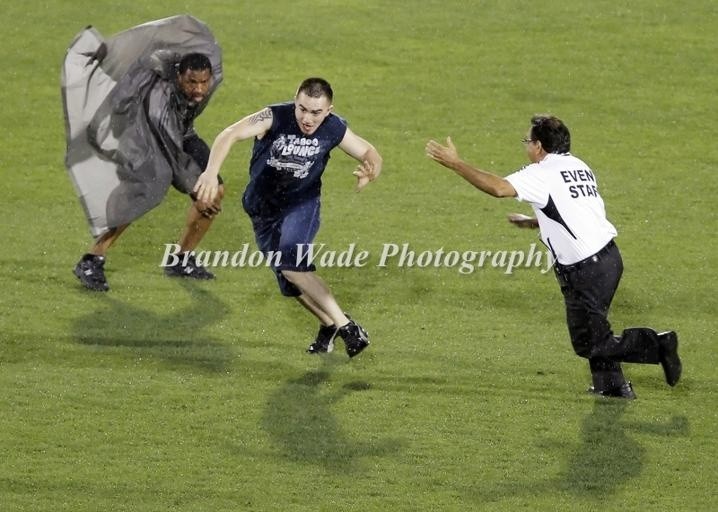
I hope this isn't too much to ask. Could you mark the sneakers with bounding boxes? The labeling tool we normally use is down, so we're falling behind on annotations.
[164,251,217,281]
[72,253,110,292]
[306,320,371,358]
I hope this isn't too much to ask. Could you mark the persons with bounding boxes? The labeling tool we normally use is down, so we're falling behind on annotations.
[424,114,682,401]
[59,14,224,292]
[193,78,384,358]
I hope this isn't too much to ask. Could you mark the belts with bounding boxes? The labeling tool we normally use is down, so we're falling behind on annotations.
[552,239,617,274]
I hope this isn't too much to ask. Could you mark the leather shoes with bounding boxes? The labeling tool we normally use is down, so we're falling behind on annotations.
[588,380,635,400]
[657,330,683,387]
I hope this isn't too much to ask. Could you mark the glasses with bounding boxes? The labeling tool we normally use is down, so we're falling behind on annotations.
[521,137,538,145]
